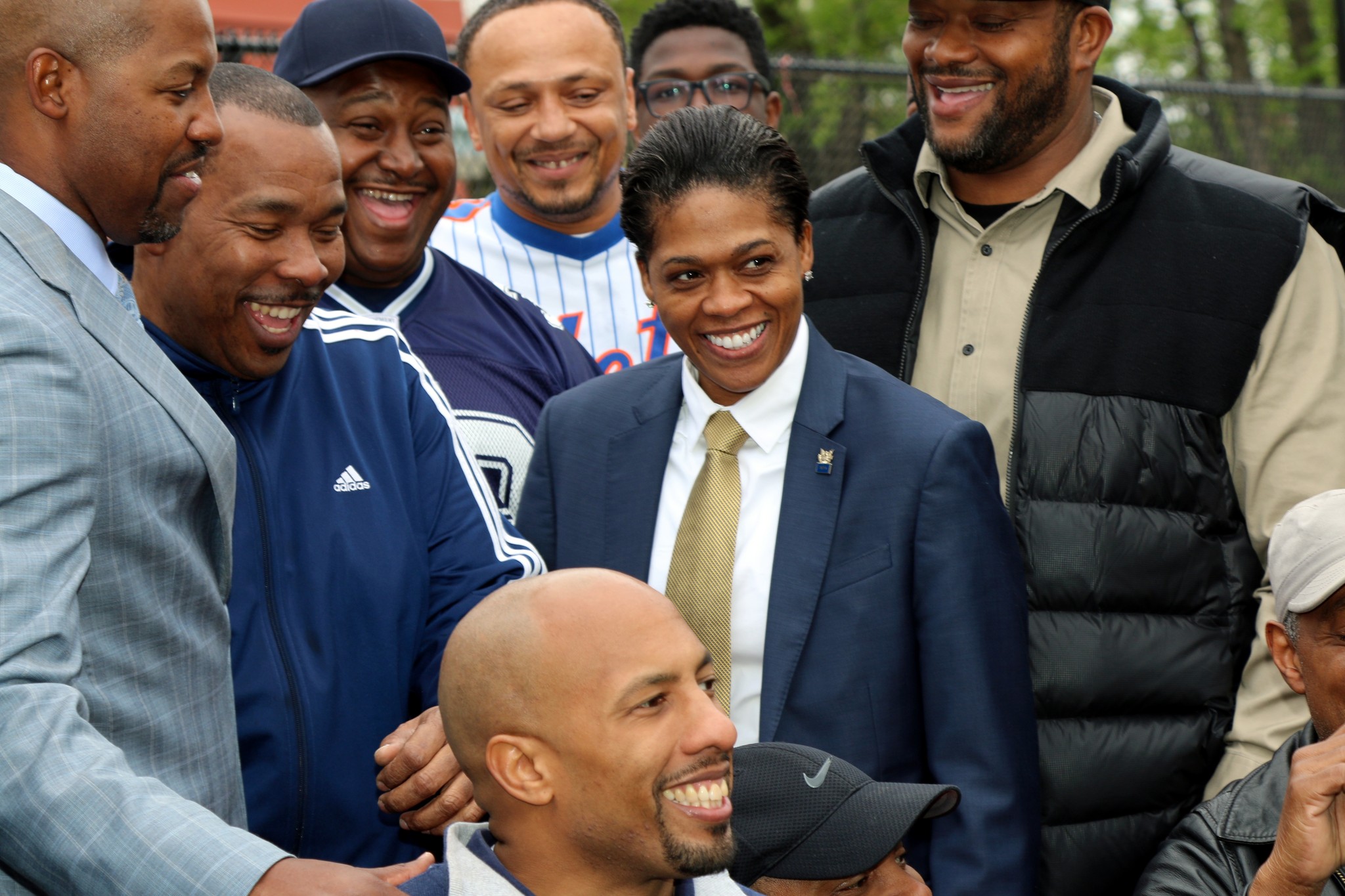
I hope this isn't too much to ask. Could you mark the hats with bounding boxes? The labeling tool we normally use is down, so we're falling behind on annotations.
[1267,488,1345,623]
[273,0,472,104]
[727,742,962,887]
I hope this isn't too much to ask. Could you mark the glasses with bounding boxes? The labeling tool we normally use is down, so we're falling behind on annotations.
[636,71,771,118]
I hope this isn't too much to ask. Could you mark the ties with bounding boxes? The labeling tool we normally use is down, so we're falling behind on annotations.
[667,410,749,718]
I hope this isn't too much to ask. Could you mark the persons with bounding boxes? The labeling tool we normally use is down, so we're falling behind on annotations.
[726,741,962,896]
[101,0,785,896]
[1135,488,1345,896]
[806,0,1345,896]
[506,109,1046,896]
[0,0,437,896]
